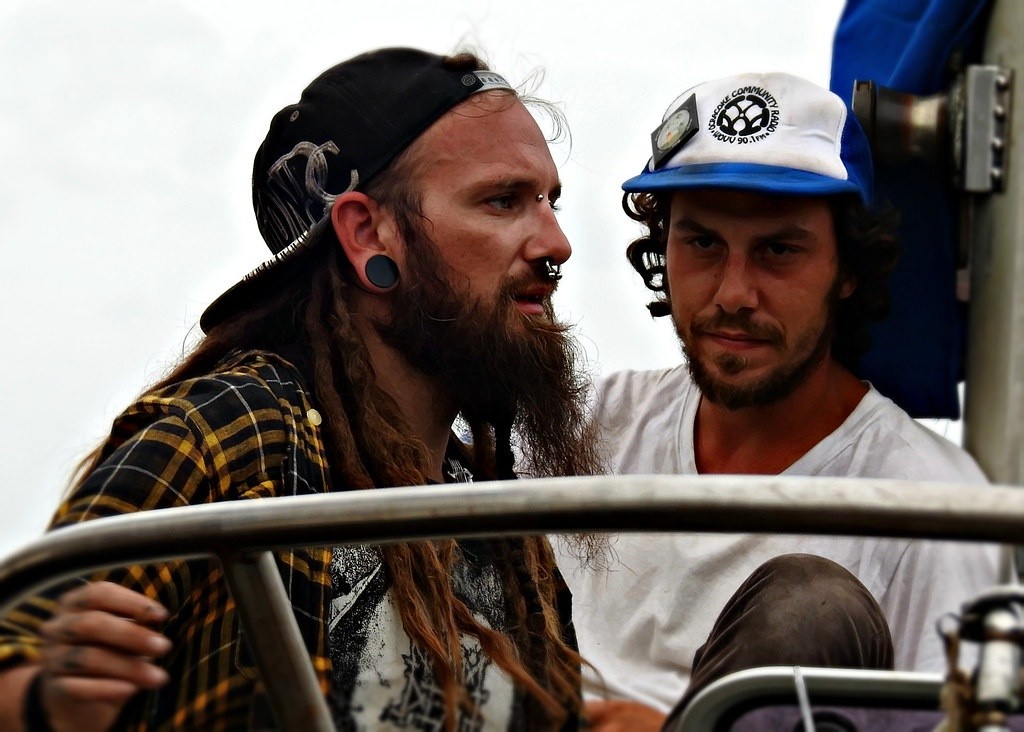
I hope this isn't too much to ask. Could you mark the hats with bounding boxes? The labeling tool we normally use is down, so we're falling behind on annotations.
[192,47,515,334]
[623,73,873,220]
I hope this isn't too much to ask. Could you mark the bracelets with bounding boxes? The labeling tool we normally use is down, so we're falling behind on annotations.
[22,675,53,732]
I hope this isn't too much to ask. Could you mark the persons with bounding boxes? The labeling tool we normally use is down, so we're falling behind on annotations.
[0,48,612,732]
[511,70,1024,732]
